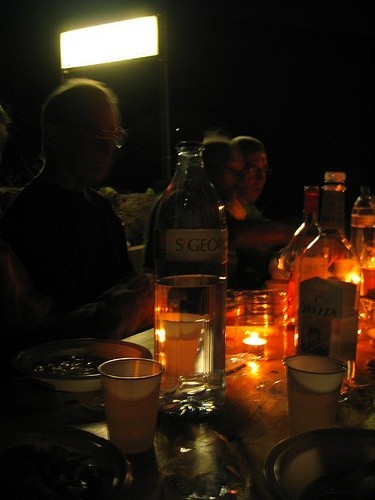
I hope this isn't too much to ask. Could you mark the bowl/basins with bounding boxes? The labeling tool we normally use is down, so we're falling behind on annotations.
[12,338,153,392]
[0,422,128,500]
[263,427,375,500]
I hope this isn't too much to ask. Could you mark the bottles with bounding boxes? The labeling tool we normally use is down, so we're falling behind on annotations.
[350,184,375,259]
[148,131,227,415]
[280,172,363,395]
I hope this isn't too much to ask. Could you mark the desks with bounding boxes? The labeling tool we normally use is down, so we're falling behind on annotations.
[74,321,375,500]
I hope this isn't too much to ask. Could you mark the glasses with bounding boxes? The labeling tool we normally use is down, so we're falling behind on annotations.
[64,123,128,149]
[218,165,246,180]
[243,166,273,177]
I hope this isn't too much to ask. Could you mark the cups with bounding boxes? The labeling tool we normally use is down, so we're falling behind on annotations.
[346,297,375,392]
[234,290,288,360]
[156,312,205,376]
[98,358,165,454]
[282,355,347,437]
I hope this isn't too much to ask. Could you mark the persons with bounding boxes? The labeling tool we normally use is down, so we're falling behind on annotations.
[143,135,338,313]
[0,87,159,349]
